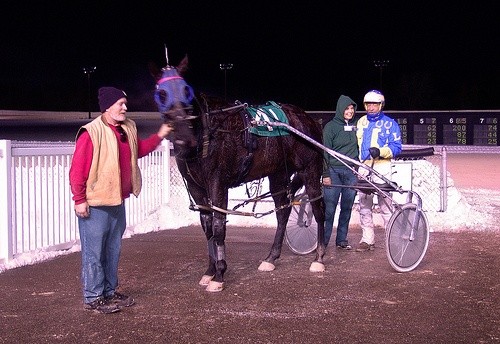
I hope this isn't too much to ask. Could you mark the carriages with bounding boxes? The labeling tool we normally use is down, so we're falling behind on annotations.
[147,44,449,292]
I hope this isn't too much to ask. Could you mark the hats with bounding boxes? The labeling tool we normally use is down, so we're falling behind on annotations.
[98,87,127,113]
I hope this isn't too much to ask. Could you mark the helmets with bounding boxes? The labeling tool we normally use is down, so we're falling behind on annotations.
[363,90,385,102]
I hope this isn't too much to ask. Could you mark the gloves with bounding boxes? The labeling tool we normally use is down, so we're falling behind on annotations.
[369,147,380,160]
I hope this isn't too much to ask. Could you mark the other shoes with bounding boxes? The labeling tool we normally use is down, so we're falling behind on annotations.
[336,245,351,249]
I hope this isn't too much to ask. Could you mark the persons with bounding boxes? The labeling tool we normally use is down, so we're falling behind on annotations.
[356,90,402,251]
[69,87,174,313]
[320,95,359,251]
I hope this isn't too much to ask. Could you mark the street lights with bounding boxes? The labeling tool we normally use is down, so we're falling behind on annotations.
[373,60,390,91]
[83,66,96,119]
[219,63,234,102]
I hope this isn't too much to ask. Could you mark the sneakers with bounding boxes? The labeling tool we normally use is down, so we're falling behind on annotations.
[356,242,375,251]
[84,291,135,313]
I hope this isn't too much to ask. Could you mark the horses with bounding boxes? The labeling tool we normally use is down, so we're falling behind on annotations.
[141,55,328,292]
